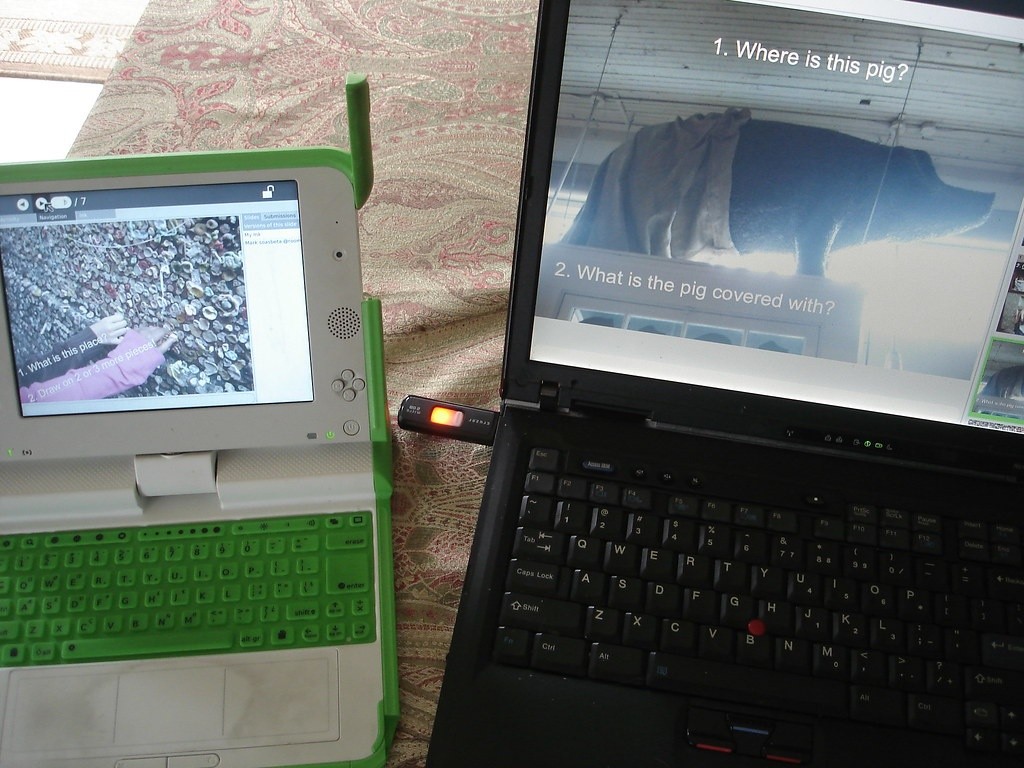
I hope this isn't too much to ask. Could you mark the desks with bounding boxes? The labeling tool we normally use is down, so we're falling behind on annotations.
[0,0,541,767]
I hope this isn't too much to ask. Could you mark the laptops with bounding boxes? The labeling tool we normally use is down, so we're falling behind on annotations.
[426,0,1024,768]
[0,71,402,768]
